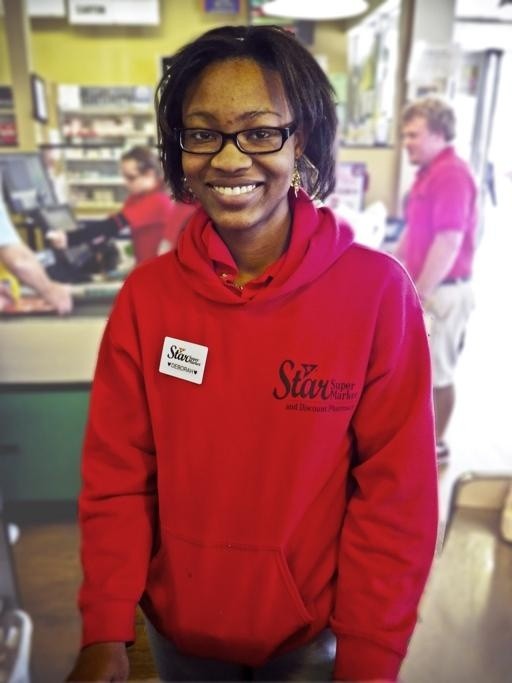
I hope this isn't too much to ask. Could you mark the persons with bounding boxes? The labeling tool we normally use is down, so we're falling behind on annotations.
[157,176,202,260]
[45,145,175,269]
[62,24,438,682]
[392,95,478,464]
[0,191,74,317]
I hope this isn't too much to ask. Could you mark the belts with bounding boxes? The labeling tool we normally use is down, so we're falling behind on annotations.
[439,273,475,289]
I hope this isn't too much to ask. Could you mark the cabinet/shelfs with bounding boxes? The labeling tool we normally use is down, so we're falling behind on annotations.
[53,79,162,214]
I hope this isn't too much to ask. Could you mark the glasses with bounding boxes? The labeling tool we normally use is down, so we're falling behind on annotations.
[173,117,299,156]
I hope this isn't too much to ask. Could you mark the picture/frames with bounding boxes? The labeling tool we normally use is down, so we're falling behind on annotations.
[32,75,48,123]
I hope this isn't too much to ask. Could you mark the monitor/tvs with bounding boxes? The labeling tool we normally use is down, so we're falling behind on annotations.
[0,151,58,217]
[32,202,96,282]
